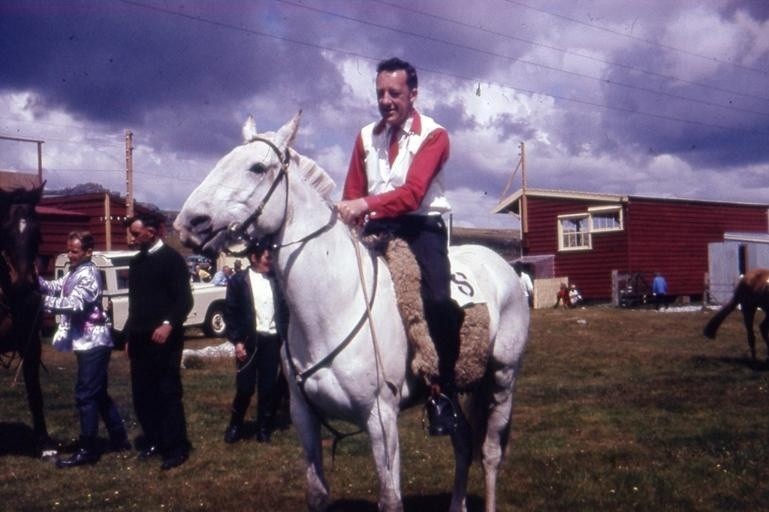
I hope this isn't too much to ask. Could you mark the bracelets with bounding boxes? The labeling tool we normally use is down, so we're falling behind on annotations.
[161,320,171,325]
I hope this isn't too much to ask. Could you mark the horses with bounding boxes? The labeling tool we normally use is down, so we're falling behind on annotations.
[171,109,531,512]
[0,180,49,447]
[704,268,769,359]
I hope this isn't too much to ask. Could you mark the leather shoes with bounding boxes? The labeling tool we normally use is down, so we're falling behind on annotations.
[56,448,90,469]
[138,443,158,460]
[258,431,271,443]
[428,398,454,436]
[225,426,240,443]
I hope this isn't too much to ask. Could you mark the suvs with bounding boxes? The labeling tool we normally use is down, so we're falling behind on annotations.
[53,251,230,346]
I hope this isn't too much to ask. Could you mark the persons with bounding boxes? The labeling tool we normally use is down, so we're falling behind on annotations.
[652,272,669,310]
[555,283,569,308]
[122,212,193,470]
[34,231,132,469]
[224,236,292,443]
[568,284,584,309]
[338,57,465,436]
[190,259,242,287]
